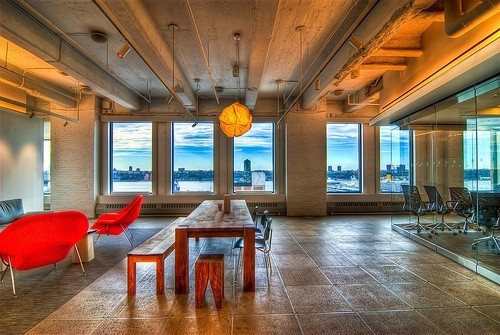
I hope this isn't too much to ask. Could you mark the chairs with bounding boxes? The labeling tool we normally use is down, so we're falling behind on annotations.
[232,205,273,287]
[401,185,500,257]
[90,194,144,247]
[0,210,90,299]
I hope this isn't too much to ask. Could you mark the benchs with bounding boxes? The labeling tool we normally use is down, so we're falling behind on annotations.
[127,217,189,297]
[0,199,24,235]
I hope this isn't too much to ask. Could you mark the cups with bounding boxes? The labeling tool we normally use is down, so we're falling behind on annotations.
[218,203,222,210]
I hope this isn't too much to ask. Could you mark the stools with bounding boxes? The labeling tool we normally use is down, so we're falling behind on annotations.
[195,254,224,310]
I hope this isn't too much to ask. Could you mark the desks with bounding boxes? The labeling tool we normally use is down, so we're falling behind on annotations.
[175,199,255,295]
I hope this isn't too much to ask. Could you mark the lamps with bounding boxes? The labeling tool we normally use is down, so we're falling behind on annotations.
[217,33,253,138]
[116,43,131,59]
[314,79,320,90]
[233,65,239,77]
